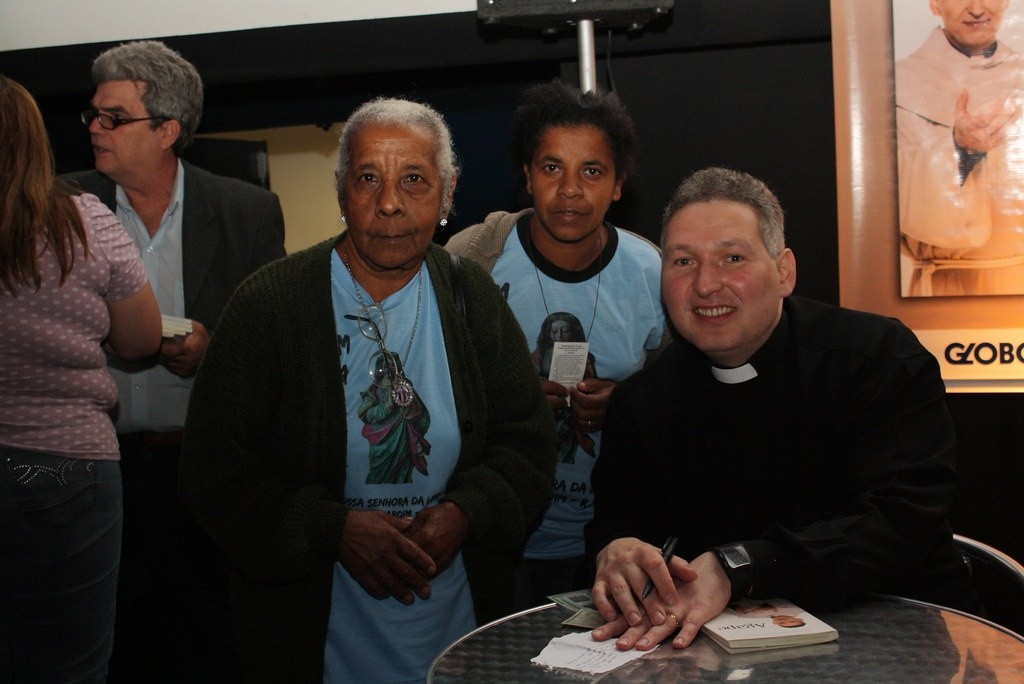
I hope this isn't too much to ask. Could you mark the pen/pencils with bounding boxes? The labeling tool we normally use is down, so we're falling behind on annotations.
[642,535,681,600]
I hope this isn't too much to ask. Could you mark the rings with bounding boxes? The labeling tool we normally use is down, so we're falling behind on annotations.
[363,552,384,563]
[670,615,679,623]
[588,421,591,426]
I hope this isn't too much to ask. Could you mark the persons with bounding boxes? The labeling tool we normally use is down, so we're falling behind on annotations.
[584,168,975,650]
[183,97,559,684]
[56,36,287,684]
[0,73,164,684]
[445,81,679,624]
[726,598,807,628]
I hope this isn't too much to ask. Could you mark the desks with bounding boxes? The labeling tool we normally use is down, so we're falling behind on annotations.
[425,587,1024,684]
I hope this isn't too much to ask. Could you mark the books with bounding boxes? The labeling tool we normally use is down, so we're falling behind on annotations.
[159,316,194,339]
[706,597,838,655]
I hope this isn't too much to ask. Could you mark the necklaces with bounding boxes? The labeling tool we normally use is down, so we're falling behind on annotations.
[524,224,604,344]
[343,242,423,405]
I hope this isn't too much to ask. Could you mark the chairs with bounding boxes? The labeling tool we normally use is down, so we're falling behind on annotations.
[917,532,1024,639]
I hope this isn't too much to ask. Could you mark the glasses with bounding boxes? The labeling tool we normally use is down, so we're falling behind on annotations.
[344,303,399,388]
[81,109,172,131]
